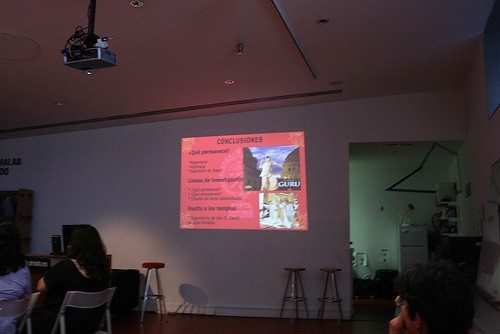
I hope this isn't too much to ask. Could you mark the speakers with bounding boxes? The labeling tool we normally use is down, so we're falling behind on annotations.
[50,235,61,255]
[110,269,140,317]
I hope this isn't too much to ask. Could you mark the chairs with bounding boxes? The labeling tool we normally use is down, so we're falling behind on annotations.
[48,287,118,334]
[0,292,42,334]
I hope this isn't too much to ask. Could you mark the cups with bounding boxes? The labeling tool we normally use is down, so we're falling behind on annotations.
[51,235,62,252]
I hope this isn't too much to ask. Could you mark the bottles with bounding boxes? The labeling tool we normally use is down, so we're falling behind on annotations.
[349,242,357,267]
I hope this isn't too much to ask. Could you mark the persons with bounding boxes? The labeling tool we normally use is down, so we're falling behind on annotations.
[37,225,111,334]
[0,221,32,334]
[389,260,476,334]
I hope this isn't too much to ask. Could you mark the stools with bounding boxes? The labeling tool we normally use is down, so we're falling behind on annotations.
[277,268,310,326]
[137,262,169,324]
[315,268,345,332]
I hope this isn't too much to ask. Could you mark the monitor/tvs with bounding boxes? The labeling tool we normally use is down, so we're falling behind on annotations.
[62,224,90,254]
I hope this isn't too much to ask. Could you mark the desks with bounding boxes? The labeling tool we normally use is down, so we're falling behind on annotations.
[22,254,112,307]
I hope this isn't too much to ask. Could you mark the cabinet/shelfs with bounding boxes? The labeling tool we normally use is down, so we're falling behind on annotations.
[437,200,483,237]
[0,189,34,249]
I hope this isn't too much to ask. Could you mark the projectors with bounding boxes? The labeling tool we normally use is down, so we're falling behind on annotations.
[63,47,118,70]
